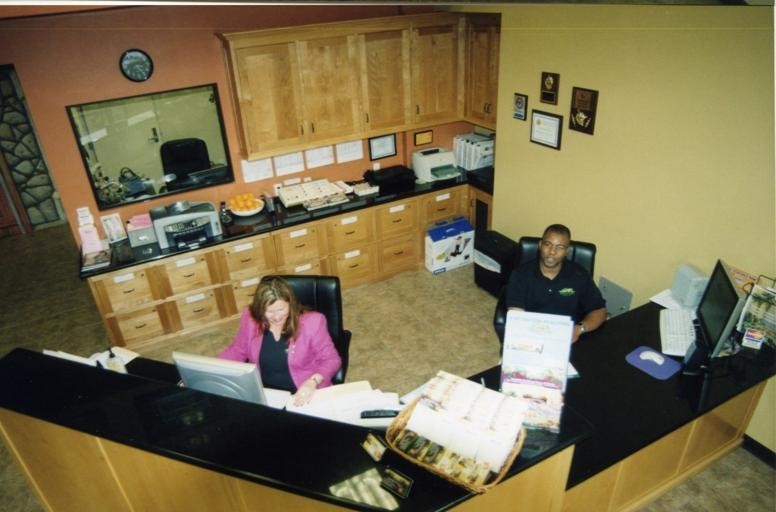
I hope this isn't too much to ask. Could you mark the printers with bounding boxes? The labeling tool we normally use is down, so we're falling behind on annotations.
[149,199,222,250]
[411,147,462,183]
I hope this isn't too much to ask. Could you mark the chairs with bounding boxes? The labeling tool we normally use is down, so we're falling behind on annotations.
[259,274,352,383]
[161,137,211,191]
[495,236,596,355]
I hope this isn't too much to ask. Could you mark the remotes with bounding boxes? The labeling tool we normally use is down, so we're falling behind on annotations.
[359,410,400,418]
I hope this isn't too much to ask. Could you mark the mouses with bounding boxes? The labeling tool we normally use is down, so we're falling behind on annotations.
[639,350,665,368]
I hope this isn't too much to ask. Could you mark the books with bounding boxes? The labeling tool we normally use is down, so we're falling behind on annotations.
[80,250,112,272]
[275,178,380,213]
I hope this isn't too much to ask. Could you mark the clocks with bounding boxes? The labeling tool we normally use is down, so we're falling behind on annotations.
[120,49,153,82]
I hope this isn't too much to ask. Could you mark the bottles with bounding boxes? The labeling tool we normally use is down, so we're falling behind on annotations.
[219,202,233,225]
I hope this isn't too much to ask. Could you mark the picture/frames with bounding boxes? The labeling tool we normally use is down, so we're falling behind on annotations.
[414,130,433,146]
[368,133,397,161]
[530,109,563,150]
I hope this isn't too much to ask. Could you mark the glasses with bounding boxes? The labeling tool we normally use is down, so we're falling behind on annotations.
[540,241,570,251]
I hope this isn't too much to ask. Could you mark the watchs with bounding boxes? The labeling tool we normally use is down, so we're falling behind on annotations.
[577,323,587,336]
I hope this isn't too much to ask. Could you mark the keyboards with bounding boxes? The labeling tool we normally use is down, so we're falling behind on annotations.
[262,388,290,410]
[659,307,697,359]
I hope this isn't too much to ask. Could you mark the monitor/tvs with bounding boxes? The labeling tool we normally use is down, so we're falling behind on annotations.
[695,260,744,358]
[171,352,268,406]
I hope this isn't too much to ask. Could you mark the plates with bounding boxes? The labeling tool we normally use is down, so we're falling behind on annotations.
[234,215,265,227]
[231,198,264,217]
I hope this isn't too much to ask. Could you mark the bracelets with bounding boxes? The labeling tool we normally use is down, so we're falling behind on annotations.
[309,375,319,387]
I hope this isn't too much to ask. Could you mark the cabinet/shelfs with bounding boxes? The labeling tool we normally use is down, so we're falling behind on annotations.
[221,20,360,162]
[460,10,500,133]
[410,13,459,130]
[359,16,411,139]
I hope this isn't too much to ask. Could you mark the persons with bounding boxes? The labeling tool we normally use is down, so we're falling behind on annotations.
[504,223,608,346]
[175,275,343,409]
